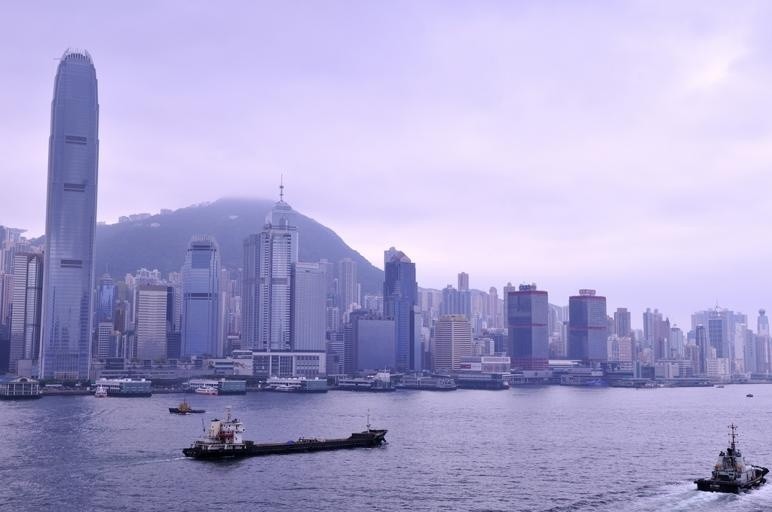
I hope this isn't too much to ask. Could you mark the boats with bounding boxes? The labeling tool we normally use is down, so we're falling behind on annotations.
[746,394,753,398]
[715,385,724,388]
[195,383,219,395]
[95,383,107,398]
[692,422,769,494]
[181,405,388,461]
[436,376,457,390]
[169,398,206,415]
[274,382,301,392]
